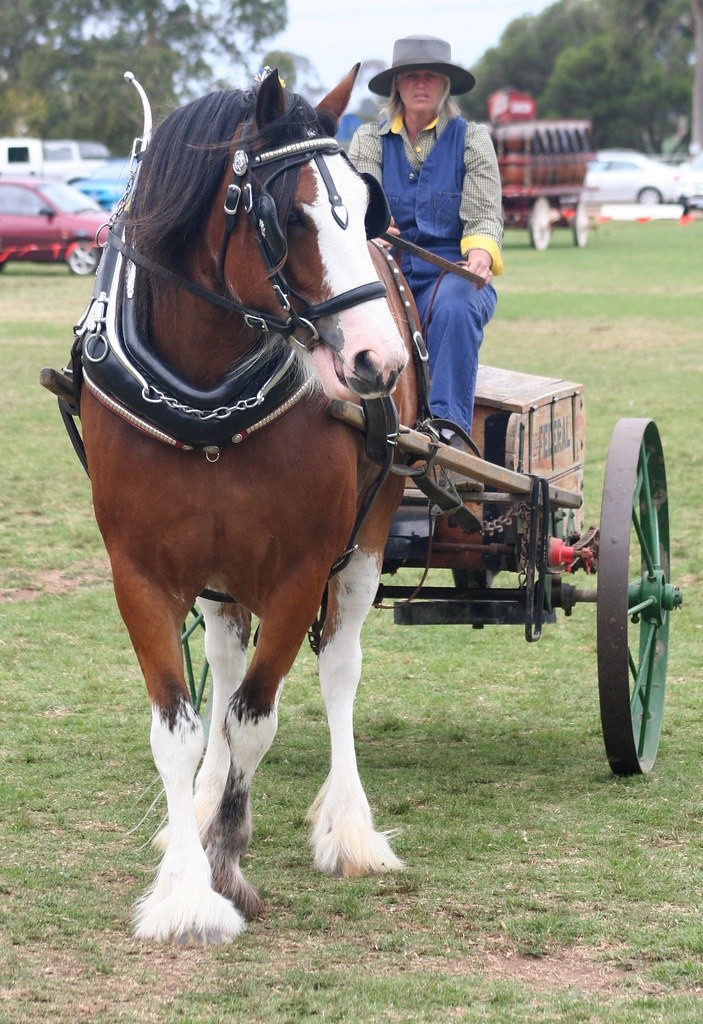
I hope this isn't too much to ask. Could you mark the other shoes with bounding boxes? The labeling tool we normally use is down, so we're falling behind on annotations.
[435,436,485,492]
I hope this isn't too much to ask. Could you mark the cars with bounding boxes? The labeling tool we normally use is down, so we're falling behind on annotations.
[583,145,703,216]
[0,136,139,276]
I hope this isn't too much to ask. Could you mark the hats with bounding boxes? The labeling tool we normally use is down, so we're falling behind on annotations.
[367,35,478,97]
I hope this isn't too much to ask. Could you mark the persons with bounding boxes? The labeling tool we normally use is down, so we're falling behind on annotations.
[342,38,505,493]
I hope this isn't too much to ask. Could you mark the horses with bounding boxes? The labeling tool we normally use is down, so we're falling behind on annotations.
[70,62,429,949]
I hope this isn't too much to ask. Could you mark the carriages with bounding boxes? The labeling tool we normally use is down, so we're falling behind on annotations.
[37,62,685,950]
